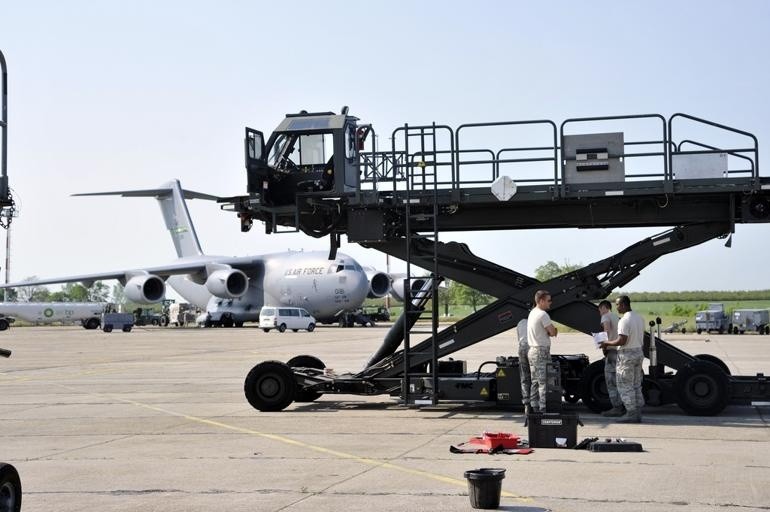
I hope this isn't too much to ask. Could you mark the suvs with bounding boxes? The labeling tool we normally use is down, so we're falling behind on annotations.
[695,303,728,334]
[134,306,160,326]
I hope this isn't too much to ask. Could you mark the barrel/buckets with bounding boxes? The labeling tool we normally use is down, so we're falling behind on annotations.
[464,468,506,509]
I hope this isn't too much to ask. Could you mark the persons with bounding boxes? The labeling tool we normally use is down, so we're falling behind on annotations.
[598,295,645,423]
[183,312,189,328]
[516,311,532,405]
[204,311,212,328]
[598,300,625,417]
[527,290,558,413]
[186,317,186,318]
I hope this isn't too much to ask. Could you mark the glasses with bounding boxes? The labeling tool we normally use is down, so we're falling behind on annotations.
[543,300,551,303]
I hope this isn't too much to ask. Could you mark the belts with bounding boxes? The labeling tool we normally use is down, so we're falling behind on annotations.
[617,348,642,352]
[530,346,550,351]
[606,350,617,353]
[520,343,527,346]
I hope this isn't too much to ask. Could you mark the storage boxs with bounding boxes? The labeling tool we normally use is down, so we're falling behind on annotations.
[526,411,578,450]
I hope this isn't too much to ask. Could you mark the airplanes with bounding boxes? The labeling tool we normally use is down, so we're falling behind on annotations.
[1,178,427,329]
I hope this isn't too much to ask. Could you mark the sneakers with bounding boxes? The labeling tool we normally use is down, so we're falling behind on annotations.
[539,408,546,413]
[615,409,641,423]
[533,407,539,413]
[601,407,624,417]
[525,405,533,416]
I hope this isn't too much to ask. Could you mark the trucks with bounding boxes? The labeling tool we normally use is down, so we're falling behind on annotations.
[731,309,770,335]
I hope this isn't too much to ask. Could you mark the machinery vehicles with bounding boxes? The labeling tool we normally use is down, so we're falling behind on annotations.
[160,298,194,327]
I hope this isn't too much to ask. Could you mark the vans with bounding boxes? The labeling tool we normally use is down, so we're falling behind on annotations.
[258,306,317,333]
